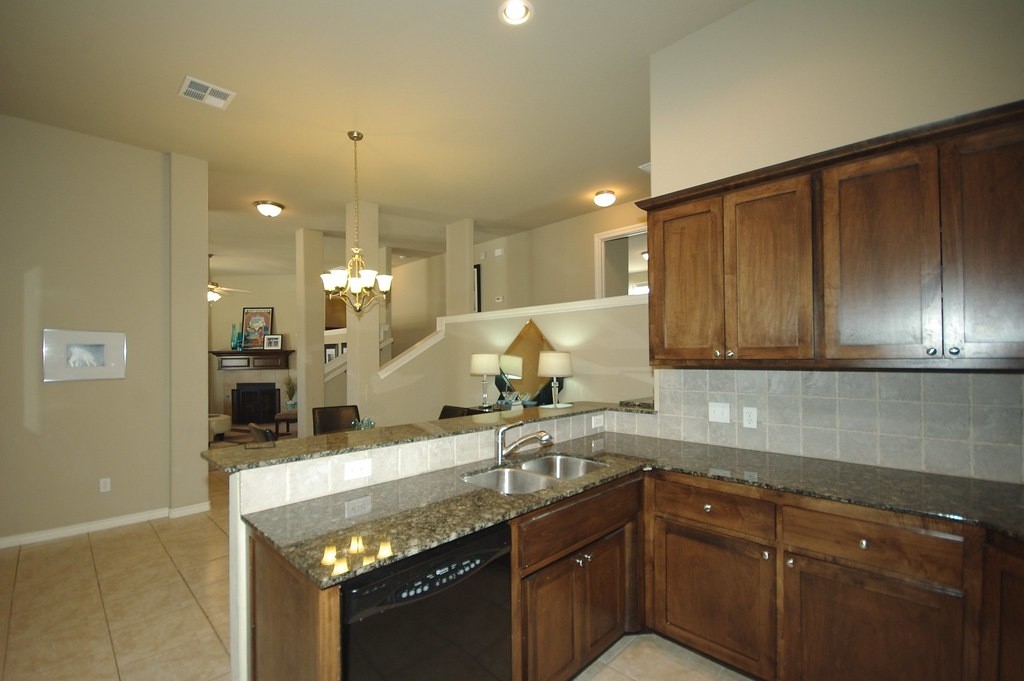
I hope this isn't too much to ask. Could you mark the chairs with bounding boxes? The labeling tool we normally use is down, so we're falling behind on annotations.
[312,405,361,435]
[248,423,276,441]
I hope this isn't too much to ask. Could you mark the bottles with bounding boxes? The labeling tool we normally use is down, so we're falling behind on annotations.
[351,415,376,430]
[231,323,243,352]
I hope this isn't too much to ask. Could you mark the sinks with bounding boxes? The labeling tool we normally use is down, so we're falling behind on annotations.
[461,464,559,496]
[518,454,612,478]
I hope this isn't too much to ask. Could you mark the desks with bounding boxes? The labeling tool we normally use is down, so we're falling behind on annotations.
[209,414,233,442]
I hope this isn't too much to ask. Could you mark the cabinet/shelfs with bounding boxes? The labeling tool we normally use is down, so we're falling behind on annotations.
[635,98,1023,368]
[510,466,642,681]
[643,466,1024,681]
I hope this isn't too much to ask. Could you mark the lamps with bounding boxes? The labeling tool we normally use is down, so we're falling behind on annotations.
[207,253,221,302]
[253,200,285,218]
[594,191,615,208]
[537,351,574,409]
[319,130,392,314]
[470,353,500,409]
[321,535,393,576]
[500,355,523,391]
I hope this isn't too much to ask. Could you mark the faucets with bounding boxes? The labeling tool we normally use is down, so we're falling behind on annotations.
[496,420,554,464]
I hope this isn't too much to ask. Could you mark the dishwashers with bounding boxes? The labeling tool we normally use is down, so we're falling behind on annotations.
[340,521,513,681]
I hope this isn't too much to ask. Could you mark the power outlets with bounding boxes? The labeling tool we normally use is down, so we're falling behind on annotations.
[708,402,730,423]
[743,407,758,428]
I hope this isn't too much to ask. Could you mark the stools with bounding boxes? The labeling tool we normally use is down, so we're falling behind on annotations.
[274,408,298,435]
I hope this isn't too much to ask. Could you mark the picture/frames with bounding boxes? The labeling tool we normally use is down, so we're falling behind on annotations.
[241,307,273,349]
[264,334,283,350]
[325,344,338,364]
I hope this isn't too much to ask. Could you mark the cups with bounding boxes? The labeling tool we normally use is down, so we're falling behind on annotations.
[502,391,518,403]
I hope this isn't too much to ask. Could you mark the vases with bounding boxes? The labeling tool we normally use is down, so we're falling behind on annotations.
[287,400,295,410]
